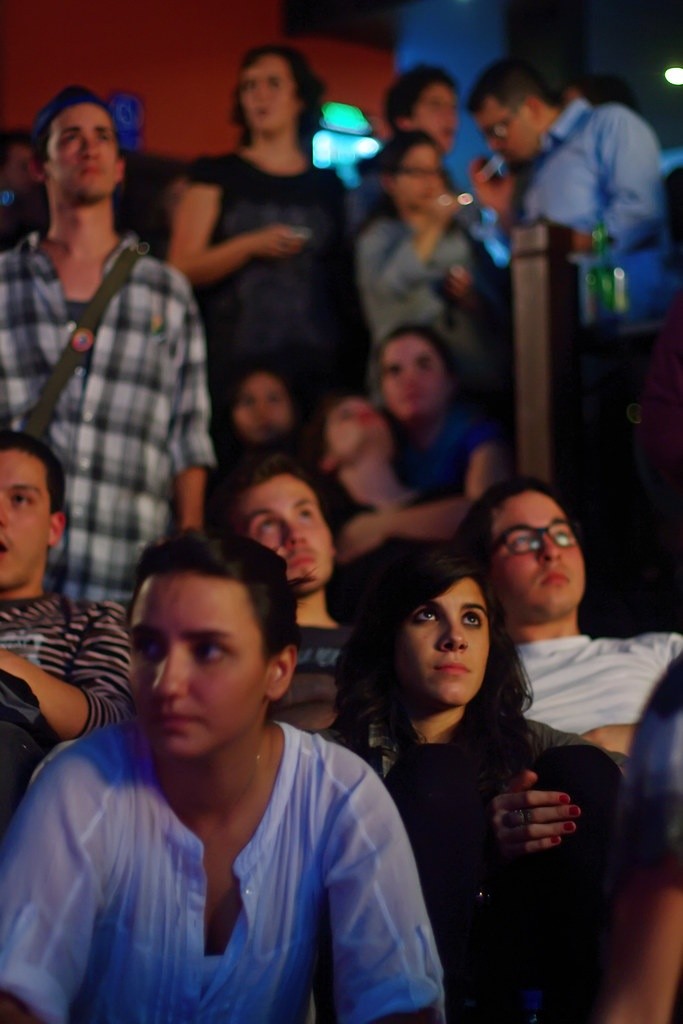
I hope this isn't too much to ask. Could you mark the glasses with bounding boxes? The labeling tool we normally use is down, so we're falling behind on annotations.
[484,101,523,139]
[492,519,583,553]
[393,167,442,178]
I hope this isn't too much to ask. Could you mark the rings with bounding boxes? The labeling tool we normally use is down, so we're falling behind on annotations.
[520,810,532,824]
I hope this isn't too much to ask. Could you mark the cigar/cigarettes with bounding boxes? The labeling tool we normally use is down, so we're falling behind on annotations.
[475,155,504,181]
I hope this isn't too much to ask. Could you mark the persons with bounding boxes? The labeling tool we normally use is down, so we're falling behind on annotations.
[0,43,683,1023]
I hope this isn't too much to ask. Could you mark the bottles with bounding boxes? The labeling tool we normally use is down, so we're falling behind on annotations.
[588,226,625,318]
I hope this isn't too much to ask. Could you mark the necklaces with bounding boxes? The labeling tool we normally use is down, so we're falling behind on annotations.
[223,754,260,818]
